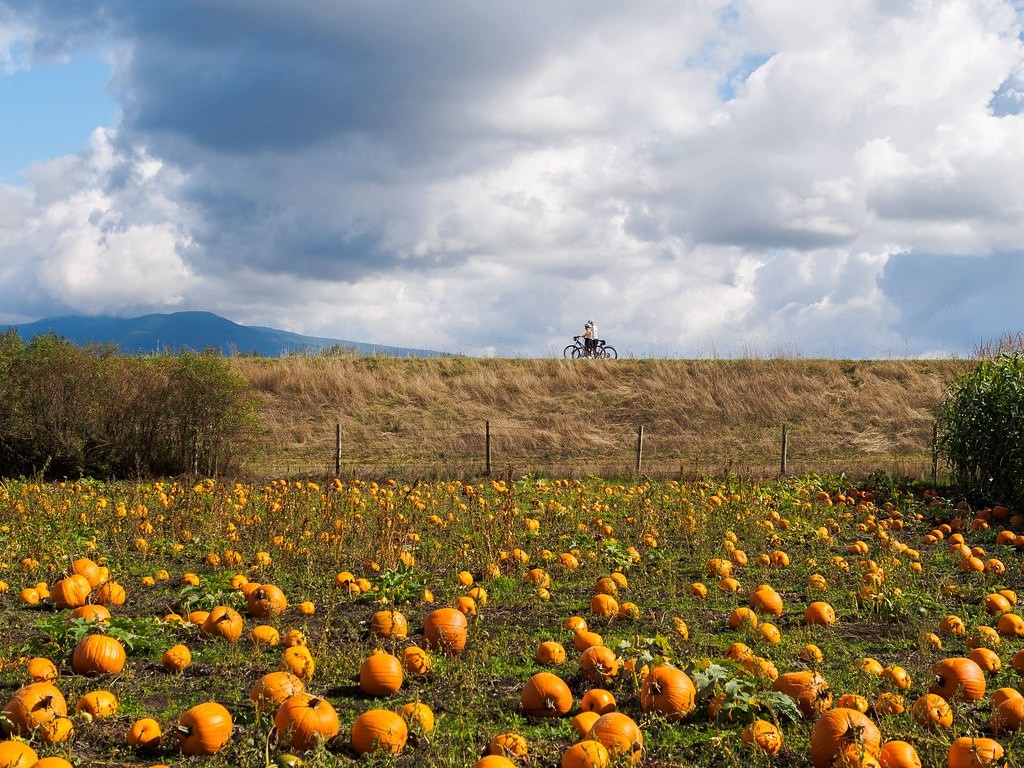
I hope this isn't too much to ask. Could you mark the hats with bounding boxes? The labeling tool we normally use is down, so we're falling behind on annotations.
[587,320,594,324]
[584,323,592,327]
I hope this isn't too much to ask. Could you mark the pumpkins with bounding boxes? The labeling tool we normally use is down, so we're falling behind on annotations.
[0,478,1024,768]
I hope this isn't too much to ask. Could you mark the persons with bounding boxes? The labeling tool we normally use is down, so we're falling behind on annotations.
[576,323,592,360]
[588,319,598,356]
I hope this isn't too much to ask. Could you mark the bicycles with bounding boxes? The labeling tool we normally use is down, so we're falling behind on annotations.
[564,336,617,360]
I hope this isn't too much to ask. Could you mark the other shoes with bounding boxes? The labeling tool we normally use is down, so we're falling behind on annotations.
[587,355,592,359]
[582,356,587,359]
